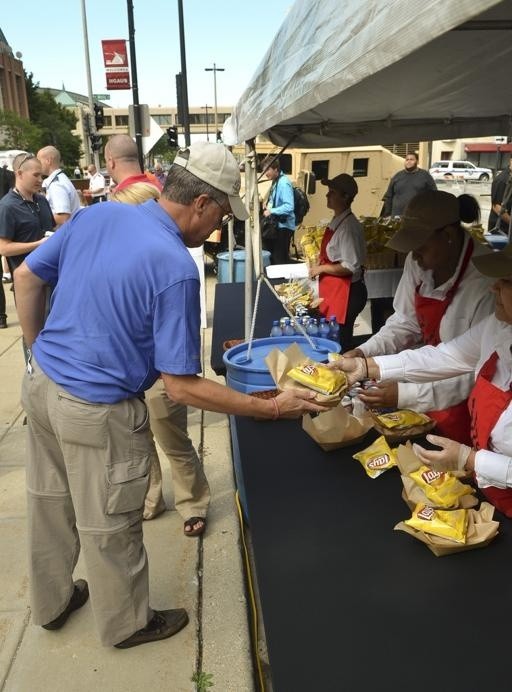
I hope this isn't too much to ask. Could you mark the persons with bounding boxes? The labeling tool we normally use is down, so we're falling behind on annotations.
[1,135,332,650]
[261,153,512,520]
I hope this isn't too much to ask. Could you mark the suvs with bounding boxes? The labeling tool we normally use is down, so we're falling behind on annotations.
[428,160,493,186]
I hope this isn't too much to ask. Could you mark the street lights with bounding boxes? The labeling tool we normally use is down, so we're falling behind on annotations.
[200,103,213,142]
[205,63,225,143]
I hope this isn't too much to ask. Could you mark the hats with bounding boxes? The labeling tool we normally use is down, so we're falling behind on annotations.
[322,174,358,194]
[173,143,249,221]
[472,242,512,278]
[385,191,460,253]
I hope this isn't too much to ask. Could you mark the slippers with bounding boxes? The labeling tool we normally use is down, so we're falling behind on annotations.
[184,517,205,536]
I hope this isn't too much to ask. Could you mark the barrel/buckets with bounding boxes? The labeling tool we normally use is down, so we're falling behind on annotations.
[222,335,342,395]
[217,250,272,283]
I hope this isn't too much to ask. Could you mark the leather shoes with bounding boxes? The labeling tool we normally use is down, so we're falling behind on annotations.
[42,579,88,629]
[113,608,188,648]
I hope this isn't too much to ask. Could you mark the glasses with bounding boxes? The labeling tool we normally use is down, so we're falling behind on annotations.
[211,197,233,226]
[18,154,35,169]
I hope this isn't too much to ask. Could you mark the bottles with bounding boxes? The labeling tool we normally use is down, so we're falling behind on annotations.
[272,315,341,341]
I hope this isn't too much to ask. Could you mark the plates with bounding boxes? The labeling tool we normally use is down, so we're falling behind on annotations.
[306,285,315,296]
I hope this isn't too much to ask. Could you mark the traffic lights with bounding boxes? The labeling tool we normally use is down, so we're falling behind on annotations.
[94,103,104,129]
[89,134,103,150]
[82,113,90,133]
[166,127,178,147]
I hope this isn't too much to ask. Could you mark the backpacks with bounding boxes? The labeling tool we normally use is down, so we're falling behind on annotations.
[294,187,309,226]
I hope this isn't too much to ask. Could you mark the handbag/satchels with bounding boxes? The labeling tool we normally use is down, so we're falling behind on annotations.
[261,214,277,240]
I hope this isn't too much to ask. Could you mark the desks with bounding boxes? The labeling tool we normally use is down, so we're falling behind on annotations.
[225,389,510,691]
[263,261,406,335]
[211,278,298,377]
